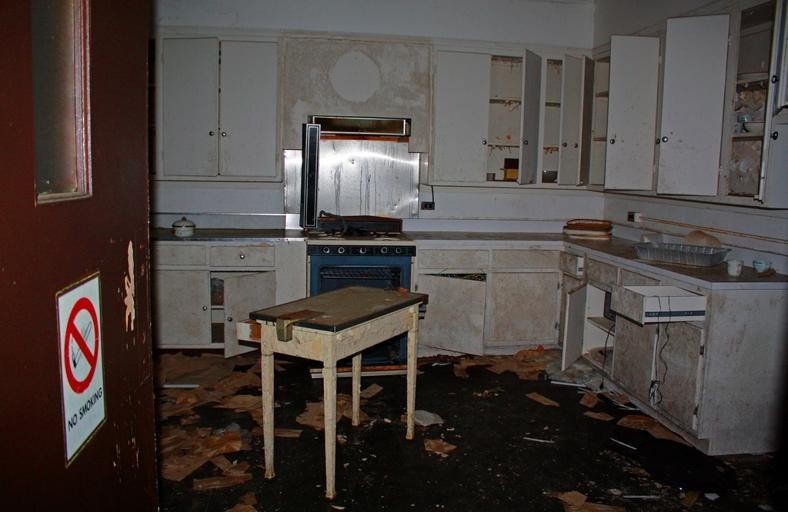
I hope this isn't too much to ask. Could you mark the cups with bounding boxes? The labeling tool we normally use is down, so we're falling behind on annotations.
[726,260,744,278]
[487,172,495,180]
[751,259,772,274]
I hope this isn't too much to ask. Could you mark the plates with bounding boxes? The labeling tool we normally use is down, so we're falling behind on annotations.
[737,71,770,80]
[561,218,613,241]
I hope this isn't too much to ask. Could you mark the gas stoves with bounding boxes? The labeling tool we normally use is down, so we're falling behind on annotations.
[307,216,411,245]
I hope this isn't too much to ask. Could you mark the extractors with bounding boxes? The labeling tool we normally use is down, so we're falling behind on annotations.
[297,113,411,141]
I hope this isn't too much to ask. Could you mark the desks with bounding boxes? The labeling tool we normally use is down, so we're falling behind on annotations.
[248,284,429,501]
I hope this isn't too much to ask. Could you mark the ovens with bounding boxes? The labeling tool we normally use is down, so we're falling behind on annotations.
[306,245,415,370]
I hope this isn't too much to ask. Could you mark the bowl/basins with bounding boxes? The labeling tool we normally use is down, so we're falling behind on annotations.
[744,121,765,133]
[171,225,195,239]
[542,170,558,182]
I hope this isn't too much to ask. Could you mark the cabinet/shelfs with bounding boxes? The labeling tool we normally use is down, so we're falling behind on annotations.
[433,44,540,188]
[154,240,277,362]
[154,26,285,181]
[416,250,562,358]
